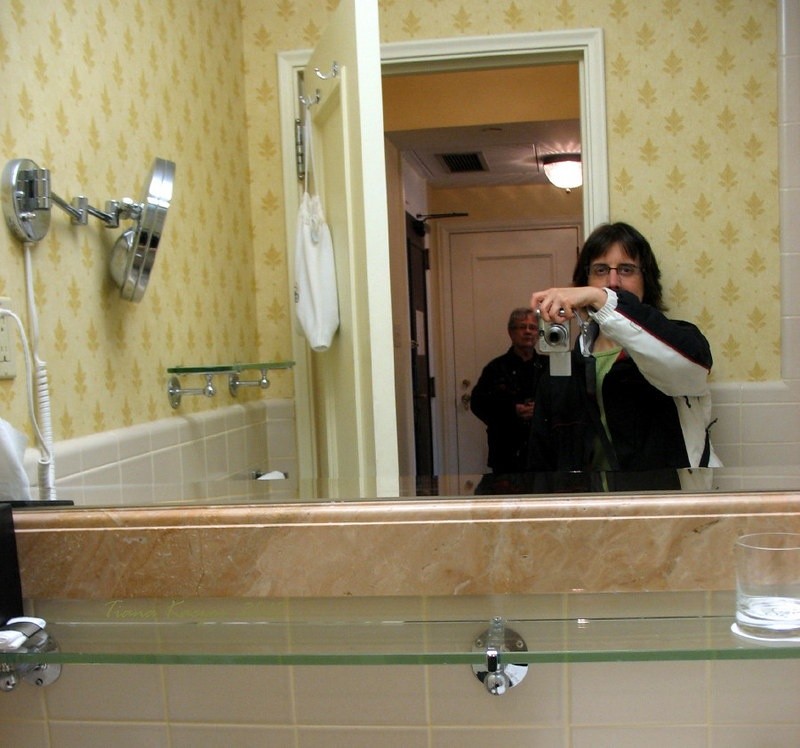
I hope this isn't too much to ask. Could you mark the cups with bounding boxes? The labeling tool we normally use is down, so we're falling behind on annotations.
[735,533,800,636]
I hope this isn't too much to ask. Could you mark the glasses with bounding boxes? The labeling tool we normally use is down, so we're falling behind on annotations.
[590,262,641,280]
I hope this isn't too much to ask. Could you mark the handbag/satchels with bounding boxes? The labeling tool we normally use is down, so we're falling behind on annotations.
[293,99,341,355]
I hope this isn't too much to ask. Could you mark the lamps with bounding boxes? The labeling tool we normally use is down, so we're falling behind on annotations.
[541,153,582,193]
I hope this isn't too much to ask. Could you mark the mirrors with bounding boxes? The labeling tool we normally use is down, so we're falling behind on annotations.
[0,0,800,511]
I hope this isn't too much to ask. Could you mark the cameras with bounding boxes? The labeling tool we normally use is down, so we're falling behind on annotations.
[535,308,570,355]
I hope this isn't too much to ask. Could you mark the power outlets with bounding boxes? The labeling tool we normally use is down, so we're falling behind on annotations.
[0,297,17,381]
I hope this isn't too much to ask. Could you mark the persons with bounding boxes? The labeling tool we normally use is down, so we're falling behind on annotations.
[470,309,547,473]
[528,222,725,490]
